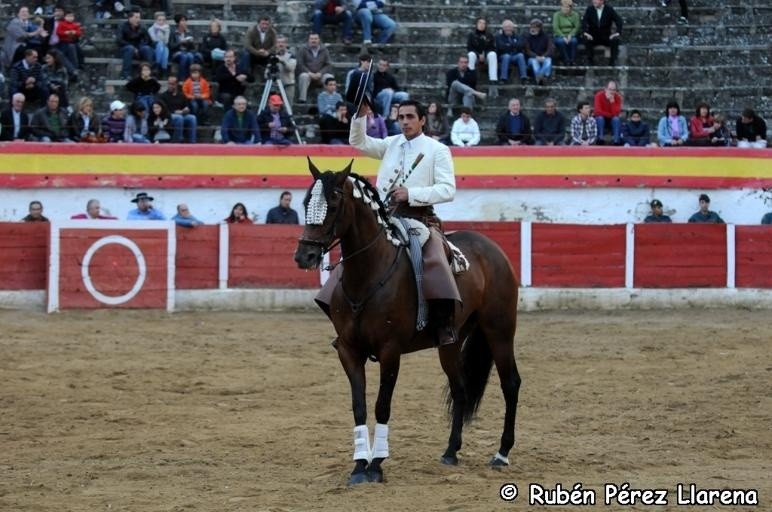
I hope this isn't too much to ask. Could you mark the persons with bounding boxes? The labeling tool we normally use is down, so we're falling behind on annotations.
[350,97,458,346]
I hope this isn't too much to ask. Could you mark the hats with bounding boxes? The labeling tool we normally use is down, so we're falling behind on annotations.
[131,191,154,203]
[269,94,284,106]
[109,100,126,112]
[344,59,376,121]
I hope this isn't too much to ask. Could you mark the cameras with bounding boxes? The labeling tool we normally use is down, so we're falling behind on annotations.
[267,53,279,66]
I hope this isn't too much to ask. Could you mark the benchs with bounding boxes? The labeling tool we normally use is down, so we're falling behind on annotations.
[0,0,771,150]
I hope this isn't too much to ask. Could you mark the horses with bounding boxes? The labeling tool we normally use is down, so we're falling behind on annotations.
[294,155,521,487]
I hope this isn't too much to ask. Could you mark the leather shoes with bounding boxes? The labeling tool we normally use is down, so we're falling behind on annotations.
[437,322,458,346]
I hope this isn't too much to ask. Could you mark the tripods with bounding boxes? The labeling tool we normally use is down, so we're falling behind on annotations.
[256,66,302,144]
